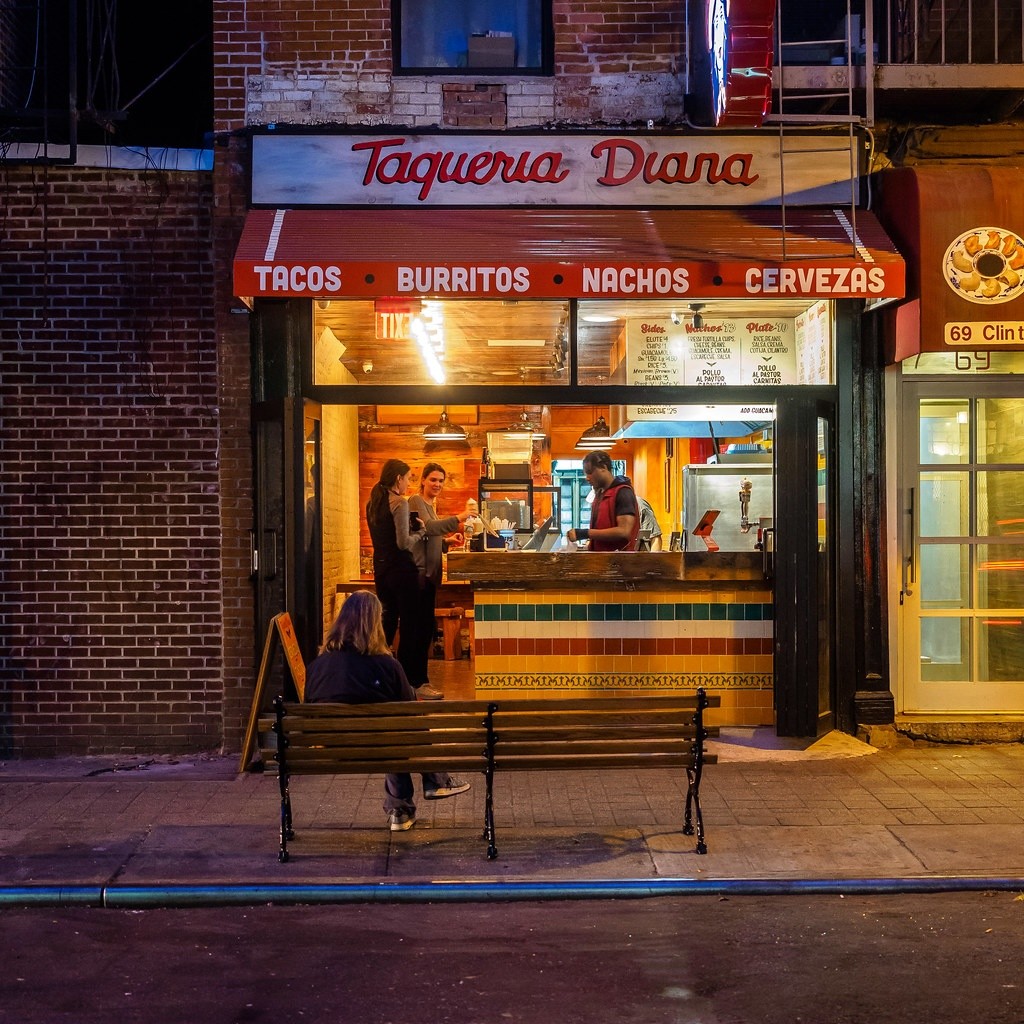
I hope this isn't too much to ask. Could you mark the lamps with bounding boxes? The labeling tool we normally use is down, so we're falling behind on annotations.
[573,405,612,450]
[425,437,466,441]
[422,406,466,437]
[688,304,705,328]
[504,436,544,440]
[581,375,621,440]
[577,377,617,446]
[503,366,547,436]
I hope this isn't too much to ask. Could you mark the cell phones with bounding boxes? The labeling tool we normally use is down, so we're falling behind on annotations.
[410,511,420,531]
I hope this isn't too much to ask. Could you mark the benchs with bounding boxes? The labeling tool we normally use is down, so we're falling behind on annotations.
[272,687,721,864]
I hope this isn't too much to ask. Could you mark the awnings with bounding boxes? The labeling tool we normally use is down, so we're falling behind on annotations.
[233,208,905,298]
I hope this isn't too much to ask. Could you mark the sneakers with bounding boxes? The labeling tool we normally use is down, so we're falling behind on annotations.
[424,778,470,800]
[386,808,416,830]
[411,683,445,699]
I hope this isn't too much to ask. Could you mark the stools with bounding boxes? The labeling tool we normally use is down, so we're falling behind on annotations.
[465,610,475,662]
[428,607,464,660]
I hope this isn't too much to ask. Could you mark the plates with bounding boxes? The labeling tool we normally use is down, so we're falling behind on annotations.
[945,229,1024,301]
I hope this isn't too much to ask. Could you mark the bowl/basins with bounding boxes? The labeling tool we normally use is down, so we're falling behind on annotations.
[499,528,516,549]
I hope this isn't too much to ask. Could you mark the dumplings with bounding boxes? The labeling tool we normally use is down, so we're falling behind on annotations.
[952,231,1024,297]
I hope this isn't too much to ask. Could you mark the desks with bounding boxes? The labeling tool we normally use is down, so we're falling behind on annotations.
[337,582,473,655]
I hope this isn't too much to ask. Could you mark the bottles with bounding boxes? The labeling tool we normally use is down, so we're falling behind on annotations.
[481,446,495,479]
[463,515,484,552]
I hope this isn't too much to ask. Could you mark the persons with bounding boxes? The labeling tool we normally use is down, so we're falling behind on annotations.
[635,496,663,552]
[366,458,445,700]
[304,590,471,832]
[566,450,639,552]
[408,462,478,617]
